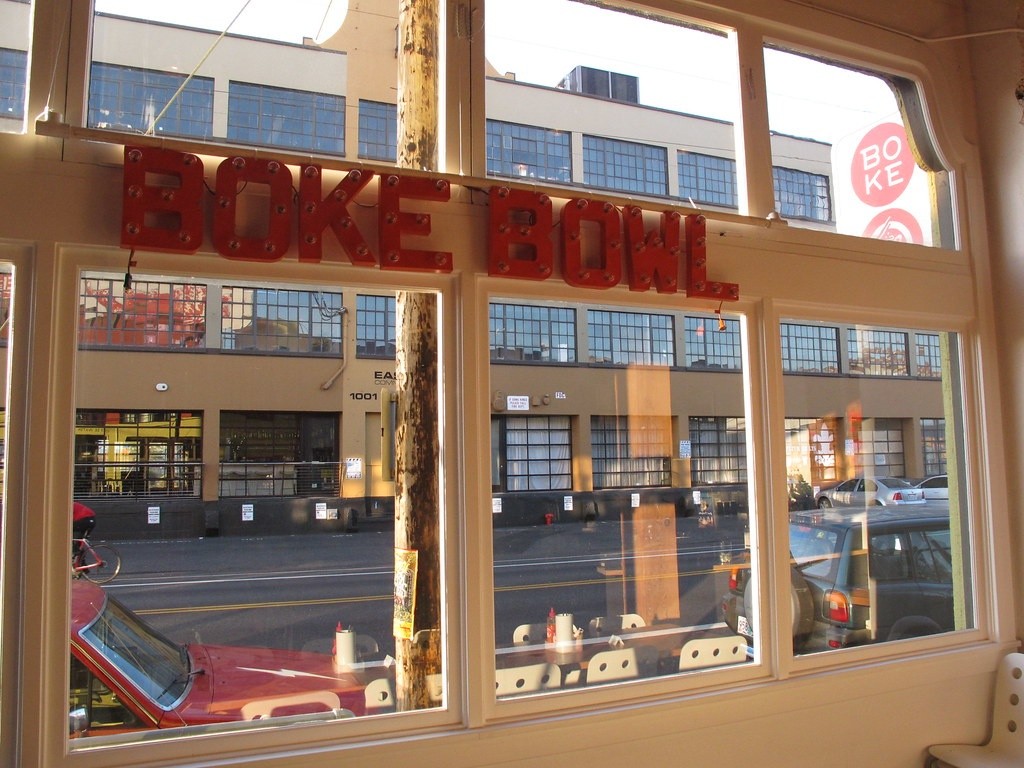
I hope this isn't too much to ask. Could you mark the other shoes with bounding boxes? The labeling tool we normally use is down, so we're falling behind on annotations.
[81,568,89,574]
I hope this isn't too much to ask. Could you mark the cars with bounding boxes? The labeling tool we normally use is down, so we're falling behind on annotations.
[814,475,926,509]
[914,473,948,507]
[68,578,378,739]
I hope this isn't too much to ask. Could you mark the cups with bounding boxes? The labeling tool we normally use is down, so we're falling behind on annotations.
[336,630,357,664]
[554,613,574,653]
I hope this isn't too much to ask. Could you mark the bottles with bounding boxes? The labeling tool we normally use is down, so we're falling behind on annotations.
[546,607,556,643]
[331,621,342,665]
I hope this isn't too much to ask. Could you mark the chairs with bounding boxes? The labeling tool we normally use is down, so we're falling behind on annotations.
[492,661,561,695]
[237,689,340,721]
[589,613,645,633]
[364,675,441,713]
[678,634,748,668]
[586,644,659,686]
[513,621,583,687]
[928,653,1024,768]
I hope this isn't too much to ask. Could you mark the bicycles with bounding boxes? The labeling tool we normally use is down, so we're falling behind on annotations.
[71,520,122,584]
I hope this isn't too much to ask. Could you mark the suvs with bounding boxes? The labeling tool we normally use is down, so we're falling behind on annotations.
[723,505,954,659]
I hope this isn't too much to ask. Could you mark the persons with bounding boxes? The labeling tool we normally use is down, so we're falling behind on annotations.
[72,501,96,573]
